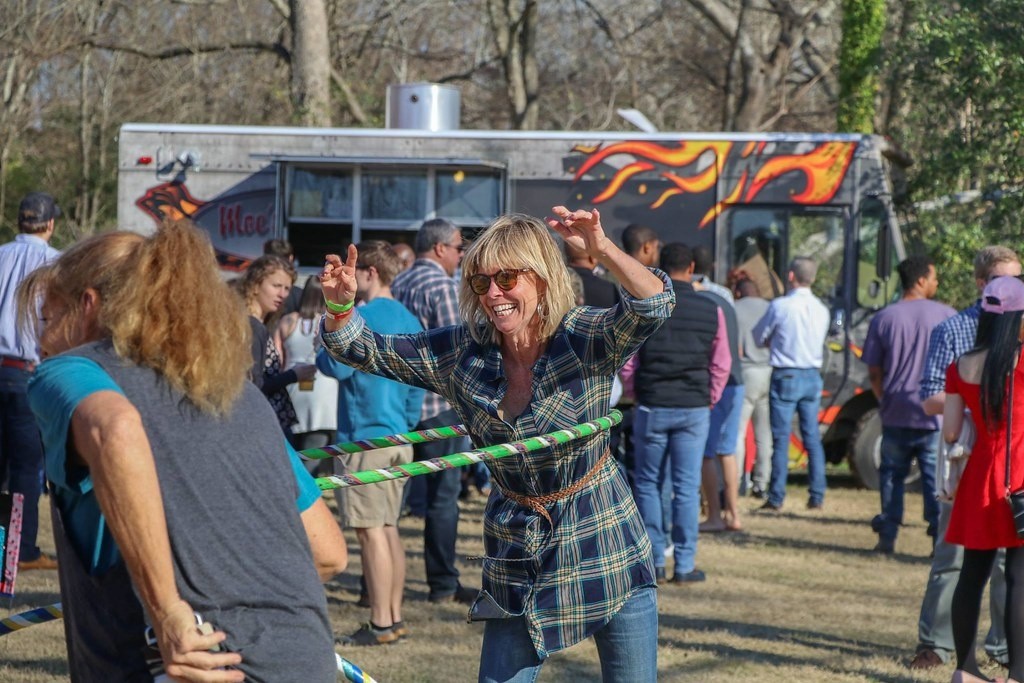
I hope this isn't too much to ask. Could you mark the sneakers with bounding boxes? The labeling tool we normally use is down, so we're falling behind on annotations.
[330,620,407,648]
[656,567,705,585]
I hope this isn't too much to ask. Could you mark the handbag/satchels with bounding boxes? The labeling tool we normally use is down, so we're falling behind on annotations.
[1005,488,1024,538]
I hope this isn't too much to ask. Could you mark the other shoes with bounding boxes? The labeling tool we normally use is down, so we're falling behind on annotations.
[754,503,780,516]
[872,533,894,556]
[806,502,823,513]
[430,589,479,605]
[910,650,941,673]
[952,667,1022,683]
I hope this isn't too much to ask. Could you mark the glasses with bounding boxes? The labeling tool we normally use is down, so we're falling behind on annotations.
[444,242,463,252]
[467,267,535,295]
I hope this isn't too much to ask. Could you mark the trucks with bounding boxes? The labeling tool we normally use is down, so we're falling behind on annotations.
[114,82,947,490]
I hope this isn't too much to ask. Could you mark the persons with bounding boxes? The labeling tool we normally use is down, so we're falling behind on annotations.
[0,185,1022,680]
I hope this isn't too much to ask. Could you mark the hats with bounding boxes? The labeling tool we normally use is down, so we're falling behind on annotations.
[18,193,60,224]
[981,275,1024,314]
